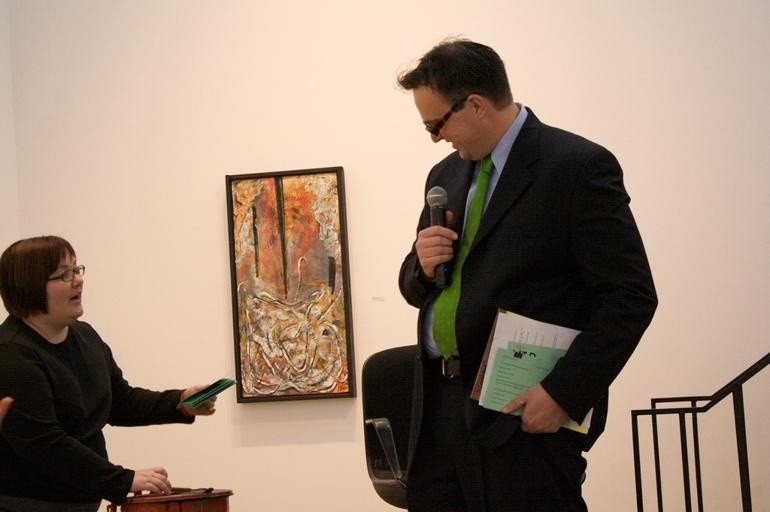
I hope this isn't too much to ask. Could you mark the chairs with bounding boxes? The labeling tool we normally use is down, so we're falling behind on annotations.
[361,344,419,509]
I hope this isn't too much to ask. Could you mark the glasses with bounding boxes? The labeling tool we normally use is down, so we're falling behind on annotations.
[425,91,485,136]
[47,265,85,282]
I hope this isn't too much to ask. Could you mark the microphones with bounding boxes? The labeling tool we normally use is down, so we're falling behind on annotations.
[427,186,451,289]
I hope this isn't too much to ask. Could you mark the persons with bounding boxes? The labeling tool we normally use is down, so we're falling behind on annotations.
[393,34,660,511]
[0,395,15,425]
[0,234,220,511]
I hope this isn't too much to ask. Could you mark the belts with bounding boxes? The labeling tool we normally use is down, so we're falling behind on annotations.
[427,355,462,375]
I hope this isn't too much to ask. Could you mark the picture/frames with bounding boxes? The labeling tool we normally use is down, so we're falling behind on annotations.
[224,166,357,404]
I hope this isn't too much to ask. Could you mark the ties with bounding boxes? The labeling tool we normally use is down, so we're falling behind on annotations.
[434,158,494,361]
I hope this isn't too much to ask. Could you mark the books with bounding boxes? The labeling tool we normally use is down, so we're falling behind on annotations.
[479,311,596,437]
[467,305,532,402]
